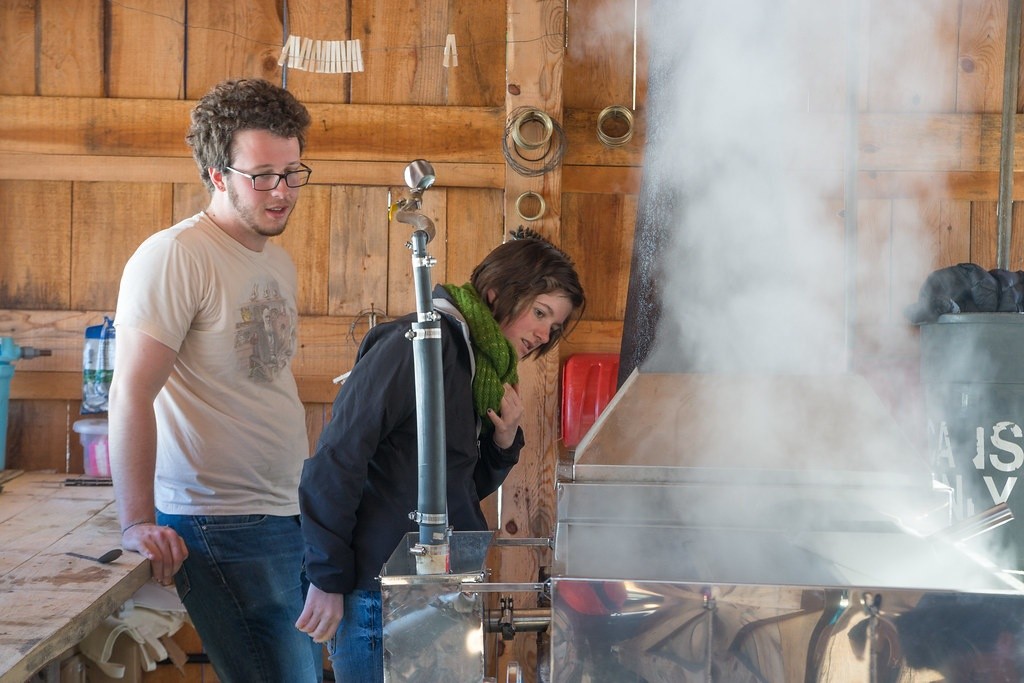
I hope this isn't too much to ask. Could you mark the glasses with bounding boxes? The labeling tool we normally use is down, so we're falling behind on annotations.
[226,162,312,191]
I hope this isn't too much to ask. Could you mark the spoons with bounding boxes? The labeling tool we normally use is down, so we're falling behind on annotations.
[67,549,122,563]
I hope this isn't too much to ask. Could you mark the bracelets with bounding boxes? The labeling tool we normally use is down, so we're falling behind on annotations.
[122,520,157,536]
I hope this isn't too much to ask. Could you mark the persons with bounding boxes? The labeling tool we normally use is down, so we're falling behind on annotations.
[294,238,587,683]
[106,77,325,683]
